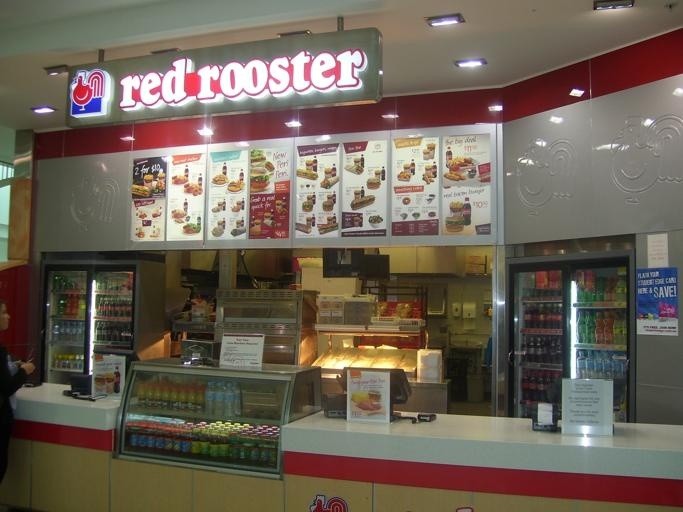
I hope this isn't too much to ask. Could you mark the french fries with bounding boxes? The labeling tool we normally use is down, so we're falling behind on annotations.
[144,175,153,183]
[158,174,165,180]
[447,156,464,166]
[324,167,332,177]
[449,199,464,217]
[306,159,312,169]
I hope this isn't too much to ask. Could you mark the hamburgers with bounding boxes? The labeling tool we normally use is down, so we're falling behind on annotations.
[250,175,270,192]
[131,184,150,197]
[252,150,266,162]
[367,177,378,189]
[323,201,333,211]
[297,169,318,180]
[303,201,314,212]
[445,216,465,233]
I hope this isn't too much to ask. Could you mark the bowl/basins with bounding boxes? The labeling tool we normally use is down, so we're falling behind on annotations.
[399,213,408,220]
[428,195,435,200]
[424,198,432,204]
[368,217,383,228]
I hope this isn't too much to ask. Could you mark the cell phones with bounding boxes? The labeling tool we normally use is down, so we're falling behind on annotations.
[418,413,436,421]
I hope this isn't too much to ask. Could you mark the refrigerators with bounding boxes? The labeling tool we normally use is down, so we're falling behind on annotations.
[566,247,636,425]
[89,261,165,397]
[38,259,89,392]
[505,252,568,426]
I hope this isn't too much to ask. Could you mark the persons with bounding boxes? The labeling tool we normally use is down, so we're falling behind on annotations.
[0,304,35,484]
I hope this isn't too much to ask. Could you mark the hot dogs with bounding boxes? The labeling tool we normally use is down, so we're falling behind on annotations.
[351,195,375,210]
[319,223,338,234]
[296,223,311,233]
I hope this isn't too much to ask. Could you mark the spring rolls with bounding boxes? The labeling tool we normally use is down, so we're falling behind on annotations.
[231,228,246,236]
[320,176,340,189]
[263,219,274,226]
[344,164,364,175]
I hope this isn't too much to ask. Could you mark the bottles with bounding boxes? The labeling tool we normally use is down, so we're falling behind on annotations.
[360,185,364,199]
[240,216,244,226]
[47,270,85,372]
[269,208,274,219]
[444,145,452,167]
[133,379,243,418]
[281,196,286,208]
[330,163,336,177]
[572,266,626,418]
[197,172,203,189]
[380,165,385,180]
[221,162,227,175]
[331,190,337,204]
[311,155,318,172]
[311,213,315,227]
[182,197,188,214]
[463,197,471,227]
[238,168,244,181]
[221,217,226,230]
[222,198,226,212]
[183,164,188,179]
[332,212,336,223]
[432,161,437,178]
[240,197,245,212]
[122,415,280,467]
[410,158,415,174]
[360,154,364,168]
[112,366,121,393]
[92,270,134,343]
[312,191,316,205]
[517,269,564,416]
[196,213,201,230]
[250,215,254,226]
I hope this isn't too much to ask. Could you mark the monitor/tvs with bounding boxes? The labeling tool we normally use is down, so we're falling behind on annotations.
[359,255,389,280]
[341,368,411,423]
[323,248,379,278]
